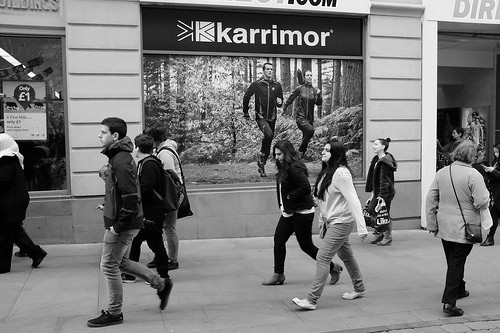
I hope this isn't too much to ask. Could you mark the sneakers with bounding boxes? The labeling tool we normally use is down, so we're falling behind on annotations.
[343,291,365,299]
[292,298,316,310]
[86,310,123,326]
[158,278,173,310]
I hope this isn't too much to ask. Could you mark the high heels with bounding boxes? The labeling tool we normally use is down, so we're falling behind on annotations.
[330,264,343,285]
[32,248,47,267]
[262,272,285,285]
[378,239,392,246]
[372,235,384,243]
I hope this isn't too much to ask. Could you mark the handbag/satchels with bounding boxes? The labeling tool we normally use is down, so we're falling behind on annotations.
[465,224,482,243]
[177,185,193,218]
[363,196,372,228]
[375,197,391,226]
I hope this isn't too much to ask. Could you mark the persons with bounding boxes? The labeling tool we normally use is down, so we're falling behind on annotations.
[292,138,368,310]
[242,62,283,175]
[147,126,185,271]
[437,127,464,165]
[86,117,174,327]
[279,69,323,161]
[0,133,48,274]
[425,140,491,316]
[121,133,169,285]
[481,143,500,247]
[14,222,29,258]
[262,139,343,285]
[365,137,397,246]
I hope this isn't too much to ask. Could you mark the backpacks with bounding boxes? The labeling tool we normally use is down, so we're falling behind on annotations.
[141,158,185,211]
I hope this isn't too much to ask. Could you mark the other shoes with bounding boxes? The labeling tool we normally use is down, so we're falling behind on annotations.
[443,303,463,316]
[457,290,469,299]
[480,239,494,246]
[258,168,267,177]
[15,250,28,257]
[147,261,156,268]
[258,153,265,167]
[121,272,136,283]
[169,262,178,270]
